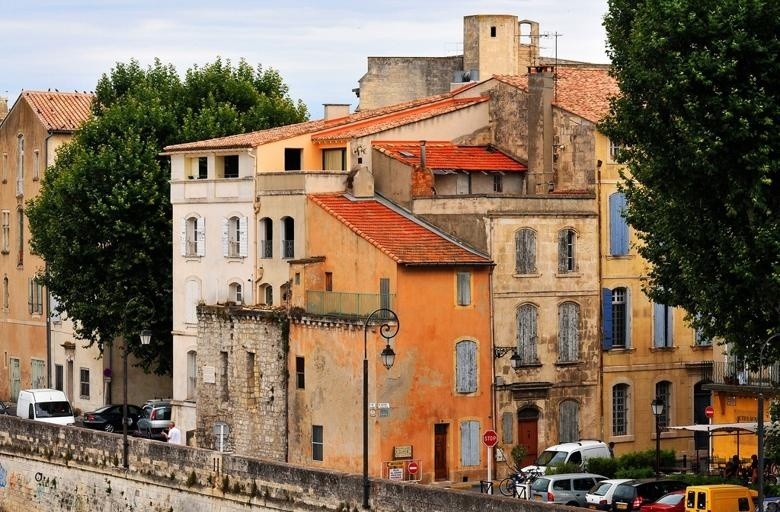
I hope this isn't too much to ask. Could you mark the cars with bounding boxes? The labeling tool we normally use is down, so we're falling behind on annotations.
[135,397,171,440]
[584,478,634,511]
[82,403,142,433]
[640,490,687,512]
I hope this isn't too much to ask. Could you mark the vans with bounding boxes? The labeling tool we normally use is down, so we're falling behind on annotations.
[515,438,612,499]
[612,478,694,512]
[15,388,75,426]
[529,472,610,506]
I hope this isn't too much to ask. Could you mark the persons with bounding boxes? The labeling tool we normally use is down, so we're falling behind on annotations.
[161,420,180,445]
[722,461,735,478]
[742,454,759,485]
[608,441,615,459]
[732,454,739,475]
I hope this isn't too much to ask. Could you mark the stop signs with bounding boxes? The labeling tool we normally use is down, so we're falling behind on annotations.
[483,431,498,448]
[705,406,714,417]
[408,463,419,474]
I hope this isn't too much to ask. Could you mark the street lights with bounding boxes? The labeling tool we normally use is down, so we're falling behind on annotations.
[650,395,665,479]
[360,307,400,512]
[122,295,155,470]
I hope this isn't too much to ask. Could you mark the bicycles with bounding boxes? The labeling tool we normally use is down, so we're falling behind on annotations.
[498,465,541,496]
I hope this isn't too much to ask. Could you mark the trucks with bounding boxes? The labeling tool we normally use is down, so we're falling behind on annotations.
[684,483,759,512]
